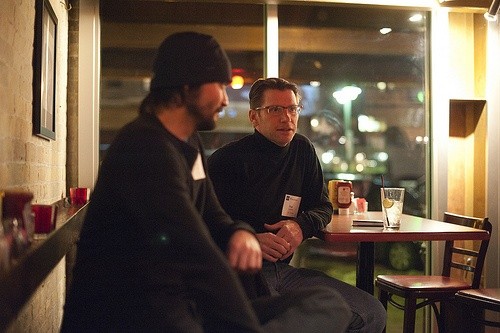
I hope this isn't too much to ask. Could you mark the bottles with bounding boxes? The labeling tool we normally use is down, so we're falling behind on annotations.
[327,180,344,215]
[2,217,23,264]
[337,181,352,215]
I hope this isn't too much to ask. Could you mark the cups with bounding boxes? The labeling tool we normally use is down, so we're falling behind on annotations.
[32,204,58,233]
[69,187,90,207]
[354,201,368,217]
[381,188,405,230]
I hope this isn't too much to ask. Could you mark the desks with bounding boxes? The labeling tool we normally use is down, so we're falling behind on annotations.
[314,212,489,296]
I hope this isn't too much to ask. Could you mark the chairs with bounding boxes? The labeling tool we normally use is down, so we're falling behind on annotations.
[375,213,493,333]
[454,288,500,333]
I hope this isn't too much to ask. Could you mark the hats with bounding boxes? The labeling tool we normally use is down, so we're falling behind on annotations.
[153,31,232,88]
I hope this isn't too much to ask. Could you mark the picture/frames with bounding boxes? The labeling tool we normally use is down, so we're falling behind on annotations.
[32,0,59,141]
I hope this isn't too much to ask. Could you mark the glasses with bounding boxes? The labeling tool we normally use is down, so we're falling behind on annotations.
[255,105,303,116]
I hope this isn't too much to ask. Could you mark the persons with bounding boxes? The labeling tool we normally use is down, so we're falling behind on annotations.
[62,31,353,333]
[207,77,387,333]
